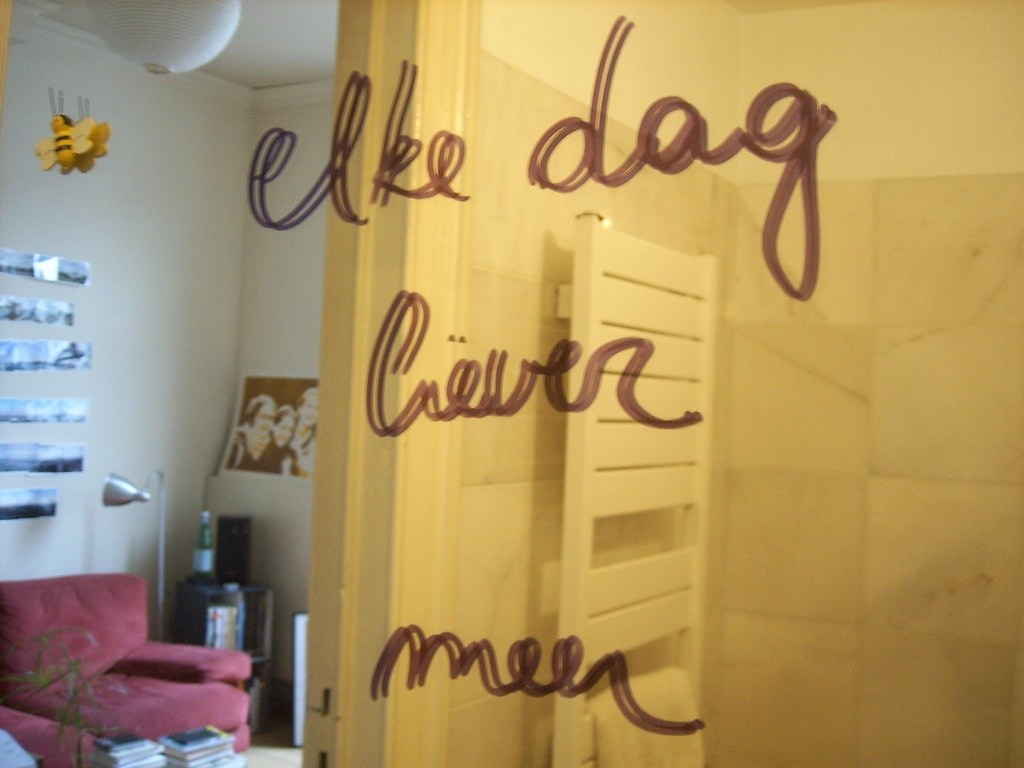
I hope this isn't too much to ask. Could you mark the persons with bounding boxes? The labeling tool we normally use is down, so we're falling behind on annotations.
[224,387,322,477]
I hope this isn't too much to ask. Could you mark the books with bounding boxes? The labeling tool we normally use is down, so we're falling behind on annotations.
[93,724,246,767]
[205,592,275,736]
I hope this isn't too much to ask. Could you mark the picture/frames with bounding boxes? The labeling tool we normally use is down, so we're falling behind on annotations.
[214,372,321,479]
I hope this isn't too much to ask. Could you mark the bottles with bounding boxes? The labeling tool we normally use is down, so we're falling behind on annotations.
[192,510,215,584]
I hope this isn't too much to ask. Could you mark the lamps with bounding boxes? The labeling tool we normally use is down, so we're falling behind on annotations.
[87,0,240,77]
[101,471,168,644]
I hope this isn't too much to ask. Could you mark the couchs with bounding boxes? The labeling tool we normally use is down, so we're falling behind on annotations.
[0,571,255,768]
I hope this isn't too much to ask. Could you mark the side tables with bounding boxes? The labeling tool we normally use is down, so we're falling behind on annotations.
[174,579,273,734]
